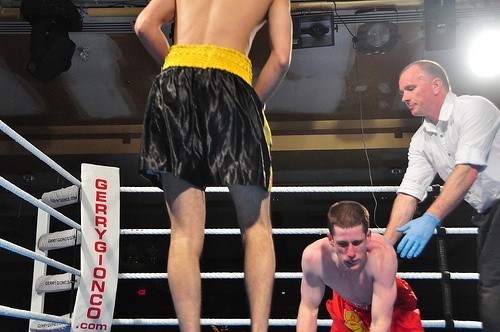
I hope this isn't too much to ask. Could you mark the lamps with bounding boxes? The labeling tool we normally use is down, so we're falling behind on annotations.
[24,23,77,85]
[291,11,335,50]
[358,22,400,56]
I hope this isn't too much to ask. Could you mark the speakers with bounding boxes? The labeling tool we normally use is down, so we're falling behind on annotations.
[20,0,83,81]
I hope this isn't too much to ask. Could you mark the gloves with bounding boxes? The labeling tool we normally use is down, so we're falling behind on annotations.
[395,213,439,259]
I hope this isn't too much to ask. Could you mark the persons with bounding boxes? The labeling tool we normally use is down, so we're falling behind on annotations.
[135,0,293,332]
[296,201,424,332]
[382,59,500,332]
